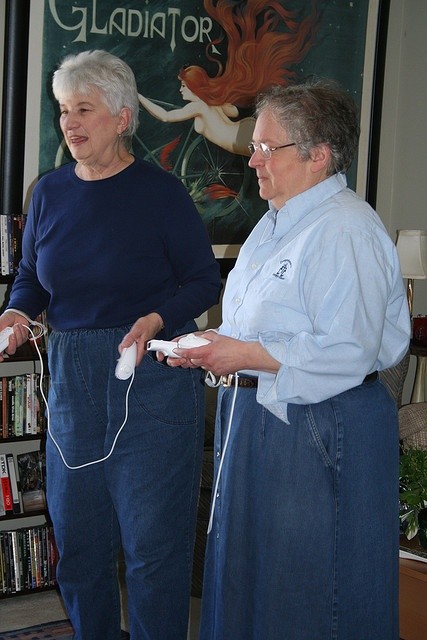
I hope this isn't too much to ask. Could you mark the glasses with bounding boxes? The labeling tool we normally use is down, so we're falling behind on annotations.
[248,142,300,159]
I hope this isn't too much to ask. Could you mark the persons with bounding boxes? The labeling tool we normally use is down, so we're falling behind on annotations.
[0,49,222,639]
[137,0,320,157]
[156,73,415,639]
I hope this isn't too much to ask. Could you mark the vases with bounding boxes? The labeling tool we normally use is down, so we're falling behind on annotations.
[418,507,427,549]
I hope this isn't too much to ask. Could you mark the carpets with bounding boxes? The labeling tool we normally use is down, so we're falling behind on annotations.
[0,618,130,640]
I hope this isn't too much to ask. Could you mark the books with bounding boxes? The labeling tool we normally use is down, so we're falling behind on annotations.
[2,452,55,515]
[6,522,74,589]
[1,213,26,281]
[1,374,61,440]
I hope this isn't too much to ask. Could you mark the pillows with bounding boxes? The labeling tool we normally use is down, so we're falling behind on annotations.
[397,400,427,452]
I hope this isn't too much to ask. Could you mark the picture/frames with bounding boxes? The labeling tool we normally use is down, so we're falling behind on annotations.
[0,0,391,278]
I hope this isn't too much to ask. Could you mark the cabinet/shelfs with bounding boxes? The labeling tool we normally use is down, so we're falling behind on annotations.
[0,275,60,598]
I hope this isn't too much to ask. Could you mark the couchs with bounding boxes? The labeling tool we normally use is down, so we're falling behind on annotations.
[192,343,427,598]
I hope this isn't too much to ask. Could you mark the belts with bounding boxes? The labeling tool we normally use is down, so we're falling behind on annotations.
[219,370,389,388]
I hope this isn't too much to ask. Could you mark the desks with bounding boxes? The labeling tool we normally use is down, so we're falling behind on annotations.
[399,534,427,640]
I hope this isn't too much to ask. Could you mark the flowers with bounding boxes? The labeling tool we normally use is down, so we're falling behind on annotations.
[398,443,427,541]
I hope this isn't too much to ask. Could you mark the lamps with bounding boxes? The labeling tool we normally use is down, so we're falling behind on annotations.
[393,229,427,339]
